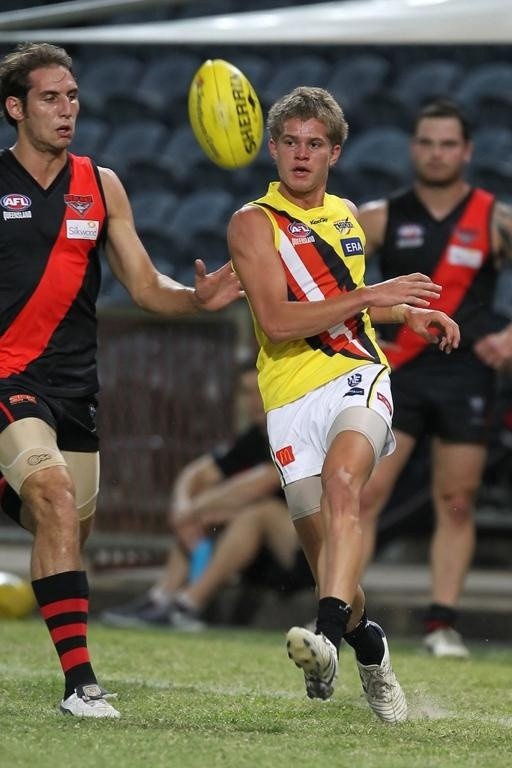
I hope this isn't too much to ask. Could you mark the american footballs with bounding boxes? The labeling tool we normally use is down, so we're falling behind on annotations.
[188,59,262,171]
[0,573,34,617]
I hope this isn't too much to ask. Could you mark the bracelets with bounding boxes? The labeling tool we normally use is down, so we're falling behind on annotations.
[390,302,408,326]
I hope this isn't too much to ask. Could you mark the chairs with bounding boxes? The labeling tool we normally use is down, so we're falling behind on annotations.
[0,48,512,320]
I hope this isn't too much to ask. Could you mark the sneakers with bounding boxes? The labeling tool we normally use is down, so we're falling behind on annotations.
[287,626,338,700]
[59,683,117,716]
[102,593,203,628]
[423,627,469,659]
[357,621,407,725]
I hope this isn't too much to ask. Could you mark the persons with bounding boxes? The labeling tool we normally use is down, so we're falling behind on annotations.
[0,41,247,722]
[101,358,317,633]
[353,105,512,666]
[223,85,462,728]
